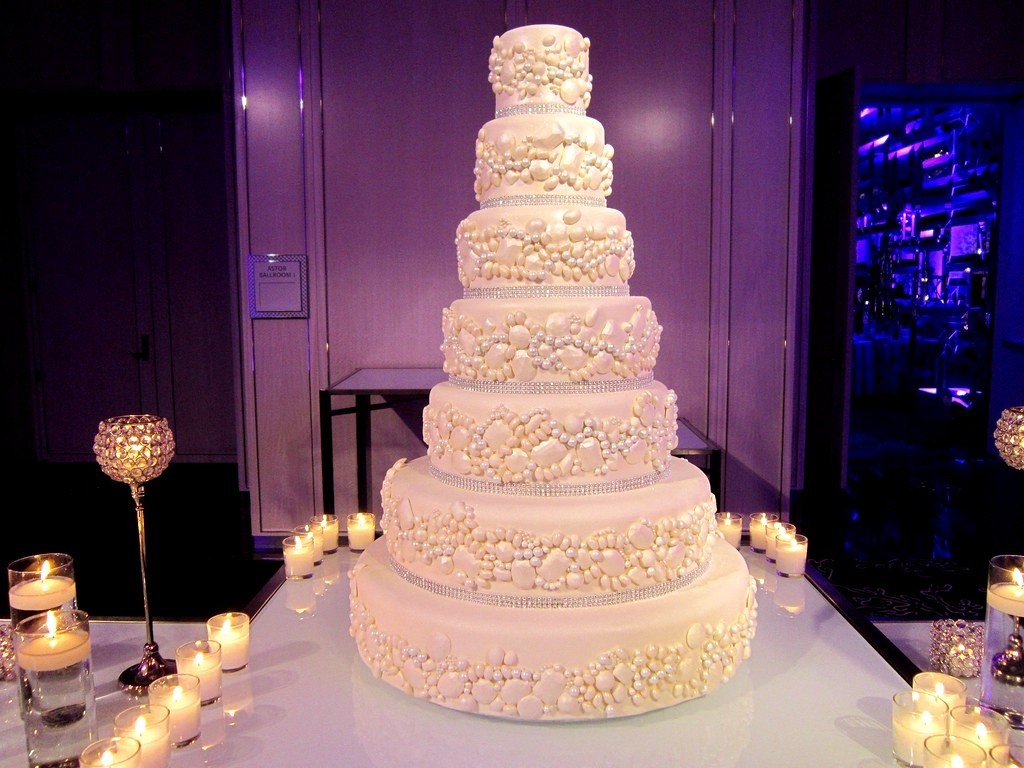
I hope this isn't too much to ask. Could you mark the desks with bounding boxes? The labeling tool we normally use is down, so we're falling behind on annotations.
[0,535,1024,768]
[673,417,721,513]
[319,366,451,516]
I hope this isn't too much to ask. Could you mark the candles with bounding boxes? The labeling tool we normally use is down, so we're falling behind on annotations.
[10,513,375,768]
[718,511,1024,768]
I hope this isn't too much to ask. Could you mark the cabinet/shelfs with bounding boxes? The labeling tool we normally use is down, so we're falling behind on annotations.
[847,97,1003,448]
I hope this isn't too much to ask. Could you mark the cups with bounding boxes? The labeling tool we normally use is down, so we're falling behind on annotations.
[79,612,250,767]
[978,555,1024,730]
[749,512,808,578]
[6,553,98,768]
[892,673,1024,768]
[347,513,376,553]
[282,514,338,580]
[716,512,742,551]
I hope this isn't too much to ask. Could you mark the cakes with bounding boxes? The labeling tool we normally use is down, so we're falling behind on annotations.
[345,25,756,721]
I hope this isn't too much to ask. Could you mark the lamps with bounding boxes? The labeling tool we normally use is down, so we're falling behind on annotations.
[992,406,1024,686]
[94,414,176,696]
